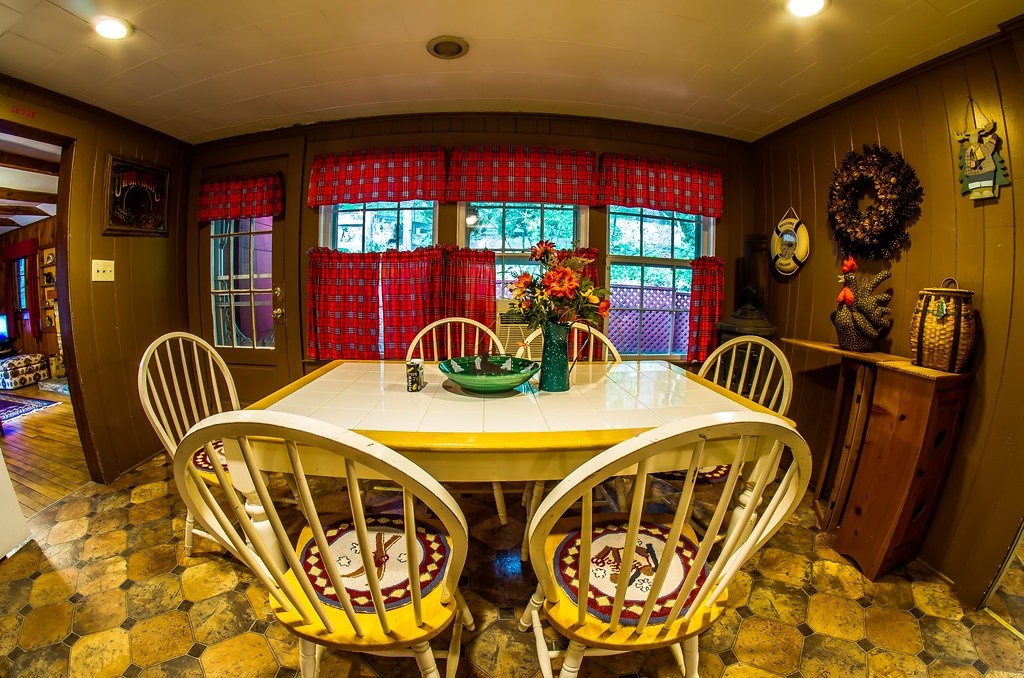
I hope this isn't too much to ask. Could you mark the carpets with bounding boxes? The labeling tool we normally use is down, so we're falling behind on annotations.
[0,392,63,424]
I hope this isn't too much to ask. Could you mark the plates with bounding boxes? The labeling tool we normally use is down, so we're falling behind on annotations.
[438,356,541,393]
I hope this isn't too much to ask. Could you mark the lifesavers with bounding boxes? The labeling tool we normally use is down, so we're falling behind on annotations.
[772,218,809,270]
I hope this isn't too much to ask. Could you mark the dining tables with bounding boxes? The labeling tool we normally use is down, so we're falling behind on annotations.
[221,359,798,568]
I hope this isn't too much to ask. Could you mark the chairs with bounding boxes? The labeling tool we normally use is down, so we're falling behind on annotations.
[642,335,794,548]
[406,317,508,527]
[137,332,240,554]
[172,410,476,678]
[514,322,629,563]
[517,411,814,678]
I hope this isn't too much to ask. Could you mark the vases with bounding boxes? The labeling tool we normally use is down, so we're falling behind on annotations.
[538,320,590,392]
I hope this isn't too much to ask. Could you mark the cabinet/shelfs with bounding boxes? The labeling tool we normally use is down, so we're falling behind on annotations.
[38,241,57,334]
[780,337,974,583]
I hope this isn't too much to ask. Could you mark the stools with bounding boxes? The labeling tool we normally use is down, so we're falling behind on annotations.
[0,353,50,390]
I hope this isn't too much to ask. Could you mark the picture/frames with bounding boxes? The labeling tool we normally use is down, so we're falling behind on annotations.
[101,151,172,238]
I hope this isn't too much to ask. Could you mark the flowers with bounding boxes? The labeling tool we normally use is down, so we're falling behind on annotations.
[506,239,615,331]
